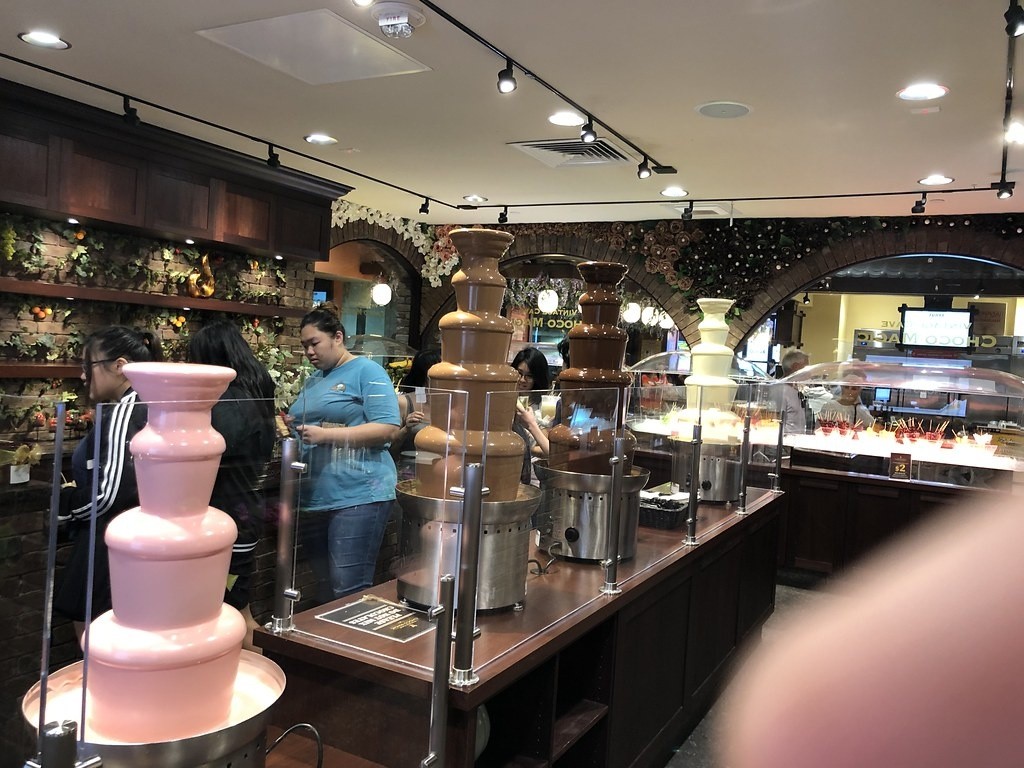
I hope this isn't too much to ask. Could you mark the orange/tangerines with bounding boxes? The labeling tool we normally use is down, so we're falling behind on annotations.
[173,316,186,327]
[32,306,52,319]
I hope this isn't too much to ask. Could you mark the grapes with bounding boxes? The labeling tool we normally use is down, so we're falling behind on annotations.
[1,224,17,260]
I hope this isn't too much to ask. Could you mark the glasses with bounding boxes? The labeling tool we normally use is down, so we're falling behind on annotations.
[843,386,858,390]
[80,359,117,372]
[520,372,534,379]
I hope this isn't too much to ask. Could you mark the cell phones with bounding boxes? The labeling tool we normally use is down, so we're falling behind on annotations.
[518,396,529,412]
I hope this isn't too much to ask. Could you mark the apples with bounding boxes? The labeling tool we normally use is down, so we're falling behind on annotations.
[73,232,85,239]
[253,261,258,268]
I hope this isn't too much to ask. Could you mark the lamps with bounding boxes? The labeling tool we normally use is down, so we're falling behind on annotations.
[996,187,1013,199]
[911,193,926,212]
[537,276,559,314]
[498,206,507,222]
[681,202,694,220]
[122,97,141,126]
[369,273,397,306]
[496,58,519,92]
[265,143,280,167]
[638,156,651,179]
[803,292,810,305]
[581,118,597,145]
[377,13,418,39]
[577,290,676,329]
[420,198,429,215]
[1004,6,1024,36]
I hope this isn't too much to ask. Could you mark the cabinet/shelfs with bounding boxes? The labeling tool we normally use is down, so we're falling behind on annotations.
[1,109,331,378]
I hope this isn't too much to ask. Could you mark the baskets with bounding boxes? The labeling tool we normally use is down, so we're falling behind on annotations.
[637,497,688,528]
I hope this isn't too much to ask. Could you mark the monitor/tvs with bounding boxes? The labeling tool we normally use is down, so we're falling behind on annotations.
[874,387,890,402]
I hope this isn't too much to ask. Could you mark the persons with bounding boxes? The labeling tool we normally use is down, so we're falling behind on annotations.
[769,359,776,375]
[287,301,400,604]
[389,347,442,479]
[820,368,874,427]
[642,363,668,409]
[57,324,165,644]
[806,379,841,413]
[188,321,276,655]
[779,349,810,433]
[511,338,576,527]
